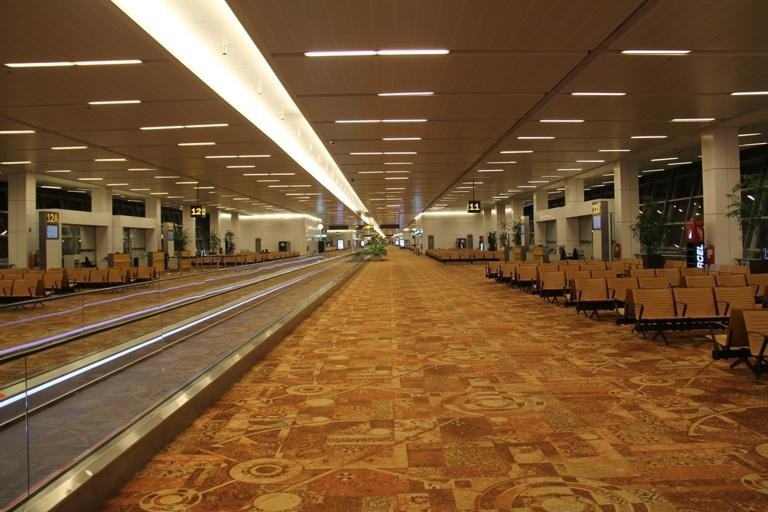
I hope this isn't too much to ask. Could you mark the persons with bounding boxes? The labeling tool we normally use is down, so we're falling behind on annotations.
[560,244,567,260]
[572,247,579,260]
[613,239,621,259]
[163,250,171,269]
[84,256,97,268]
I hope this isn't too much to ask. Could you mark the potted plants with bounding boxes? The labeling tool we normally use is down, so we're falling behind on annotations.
[725,174,768,274]
[367,239,387,261]
[628,197,667,269]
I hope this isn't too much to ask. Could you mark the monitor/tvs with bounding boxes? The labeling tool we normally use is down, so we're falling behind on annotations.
[521,223,525,235]
[592,214,601,230]
[168,231,175,240]
[46,223,59,240]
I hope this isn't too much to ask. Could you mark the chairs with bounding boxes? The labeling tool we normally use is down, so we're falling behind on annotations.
[484,260,625,294]
[0,267,157,312]
[614,287,763,347]
[194,252,298,267]
[705,305,768,380]
[426,249,500,266]
[539,265,767,322]
